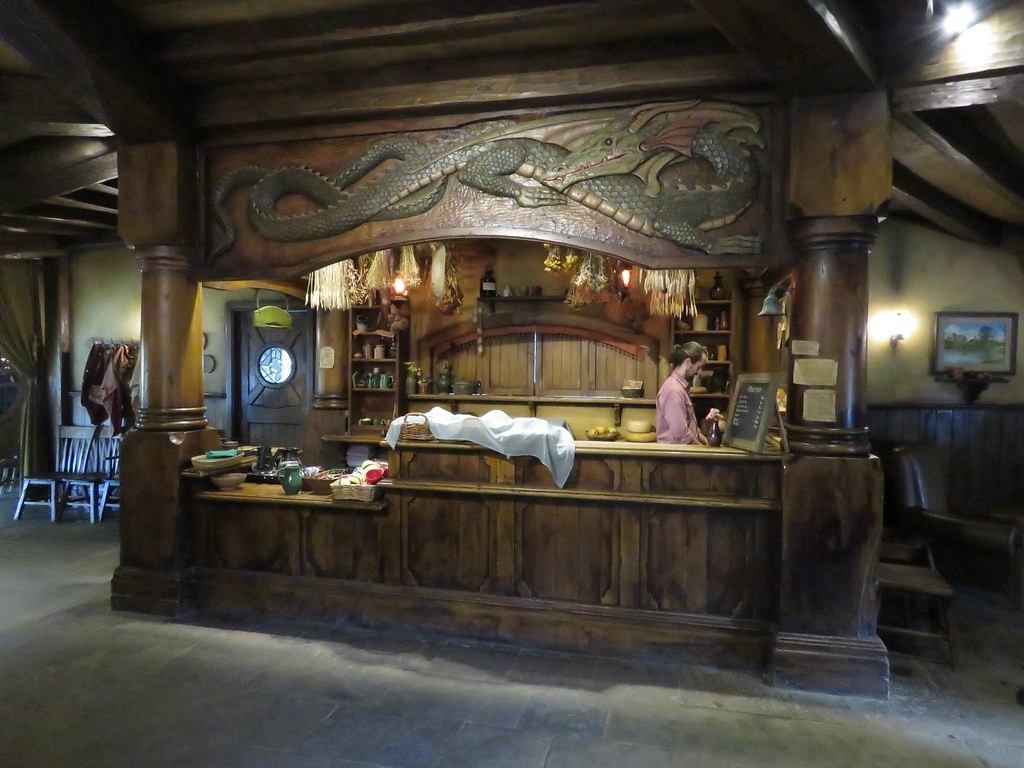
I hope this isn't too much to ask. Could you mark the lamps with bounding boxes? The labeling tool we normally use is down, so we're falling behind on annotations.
[758,286,794,316]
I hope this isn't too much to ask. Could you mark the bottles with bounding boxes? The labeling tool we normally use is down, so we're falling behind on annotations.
[374,345,384,359]
[707,412,722,447]
[481,263,497,297]
[368,368,380,388]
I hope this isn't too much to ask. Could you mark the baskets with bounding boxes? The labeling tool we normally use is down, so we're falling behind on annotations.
[305,469,347,495]
[191,450,244,471]
[330,473,383,503]
[400,413,433,440]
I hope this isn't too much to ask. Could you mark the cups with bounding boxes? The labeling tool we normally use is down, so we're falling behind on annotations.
[718,346,726,361]
[379,374,395,389]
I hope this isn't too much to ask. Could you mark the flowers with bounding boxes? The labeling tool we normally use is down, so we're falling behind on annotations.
[543,244,582,275]
[403,360,422,375]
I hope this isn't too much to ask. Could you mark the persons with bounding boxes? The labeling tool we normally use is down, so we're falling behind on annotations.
[656,341,707,445]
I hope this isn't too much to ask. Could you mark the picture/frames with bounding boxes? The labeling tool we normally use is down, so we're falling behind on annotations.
[931,312,1018,378]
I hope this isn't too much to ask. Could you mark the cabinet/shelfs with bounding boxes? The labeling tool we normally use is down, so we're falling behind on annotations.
[348,304,409,436]
[669,288,743,411]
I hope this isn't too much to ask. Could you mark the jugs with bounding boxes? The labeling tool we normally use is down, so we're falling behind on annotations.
[251,447,302,474]
[277,466,302,495]
[451,380,482,395]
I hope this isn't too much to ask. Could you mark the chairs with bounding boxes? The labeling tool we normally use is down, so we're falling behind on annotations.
[13,425,127,524]
[872,442,1024,672]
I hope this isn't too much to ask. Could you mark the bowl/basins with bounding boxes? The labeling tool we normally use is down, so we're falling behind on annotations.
[349,459,388,486]
[621,388,644,398]
[210,473,246,490]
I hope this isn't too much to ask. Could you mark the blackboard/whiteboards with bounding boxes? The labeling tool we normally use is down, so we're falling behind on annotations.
[721,373,779,454]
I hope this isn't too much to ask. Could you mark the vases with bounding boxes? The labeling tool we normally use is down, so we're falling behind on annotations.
[405,372,417,394]
